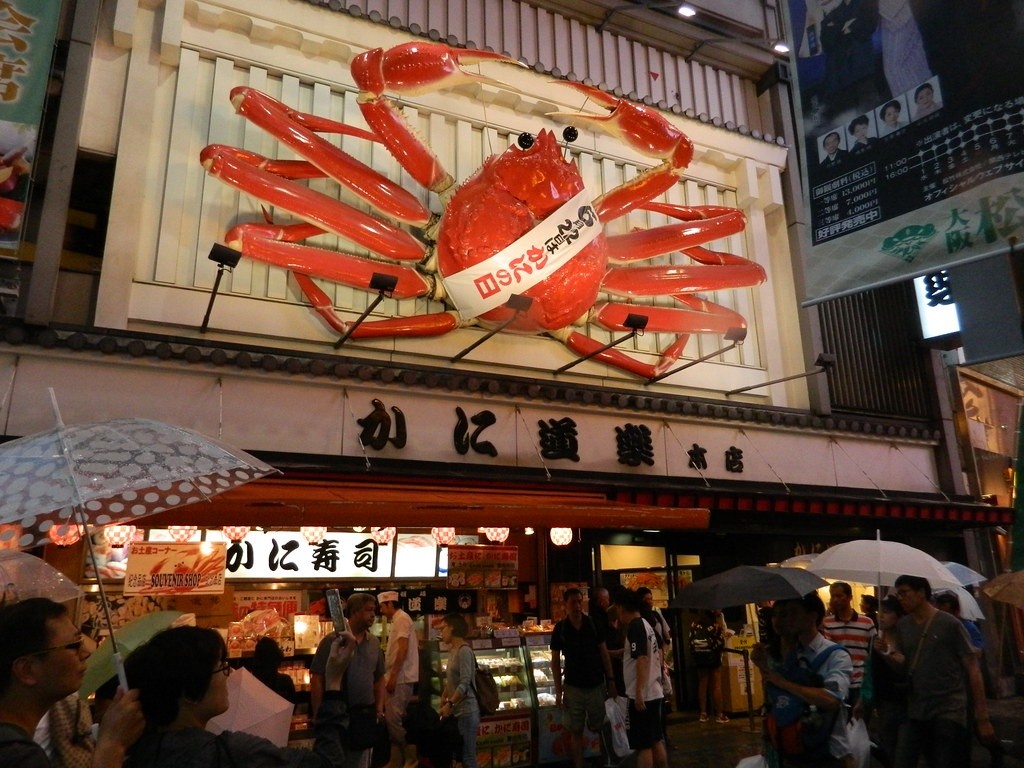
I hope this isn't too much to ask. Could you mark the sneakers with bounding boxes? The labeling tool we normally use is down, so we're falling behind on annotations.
[699,714,711,722]
[716,715,731,723]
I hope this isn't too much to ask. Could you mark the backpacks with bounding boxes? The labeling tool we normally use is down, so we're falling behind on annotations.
[689,625,721,668]
[766,644,851,768]
[458,644,500,715]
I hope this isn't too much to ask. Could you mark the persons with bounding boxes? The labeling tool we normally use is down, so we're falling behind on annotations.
[687,608,730,724]
[311,593,387,768]
[810,0,1024,165]
[749,574,994,768]
[0,597,356,768]
[438,614,481,768]
[550,585,673,768]
[377,591,420,768]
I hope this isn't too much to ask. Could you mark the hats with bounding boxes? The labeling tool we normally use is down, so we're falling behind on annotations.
[880,595,902,616]
[377,591,399,603]
[430,618,442,628]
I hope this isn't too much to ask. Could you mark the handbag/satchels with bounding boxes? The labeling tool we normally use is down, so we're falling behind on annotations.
[601,697,637,768]
[373,717,389,765]
[882,673,914,706]
[845,717,872,768]
[861,635,879,703]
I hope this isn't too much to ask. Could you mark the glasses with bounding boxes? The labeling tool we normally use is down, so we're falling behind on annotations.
[209,659,231,677]
[894,589,914,597]
[439,625,447,631]
[26,635,84,656]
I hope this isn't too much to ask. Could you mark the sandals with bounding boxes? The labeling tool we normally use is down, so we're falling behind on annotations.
[669,743,678,750]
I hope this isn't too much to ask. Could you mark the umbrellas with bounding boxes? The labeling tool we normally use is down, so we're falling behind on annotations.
[0,548,86,603]
[206,667,294,747]
[80,611,180,700]
[0,386,284,695]
[676,529,1023,656]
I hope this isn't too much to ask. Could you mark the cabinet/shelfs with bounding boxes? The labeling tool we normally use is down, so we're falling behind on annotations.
[420,632,604,768]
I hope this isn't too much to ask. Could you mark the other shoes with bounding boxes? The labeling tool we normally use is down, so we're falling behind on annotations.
[991,746,1008,768]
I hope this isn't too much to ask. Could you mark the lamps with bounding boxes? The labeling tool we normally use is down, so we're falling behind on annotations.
[596,0,698,34]
[450,294,533,363]
[686,36,790,64]
[645,326,749,386]
[552,313,649,375]
[725,353,838,397]
[335,272,398,350]
[200,243,244,334]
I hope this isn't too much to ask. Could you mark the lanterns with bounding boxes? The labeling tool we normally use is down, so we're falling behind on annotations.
[371,527,396,544]
[223,526,250,543]
[486,527,509,543]
[168,525,197,542]
[301,527,327,545]
[431,527,456,547]
[48,525,83,545]
[104,525,135,547]
[550,527,572,546]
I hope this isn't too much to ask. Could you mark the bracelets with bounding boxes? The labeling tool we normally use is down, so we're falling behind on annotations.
[448,701,454,707]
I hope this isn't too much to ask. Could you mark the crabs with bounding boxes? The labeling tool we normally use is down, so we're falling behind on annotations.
[201,44,765,376]
